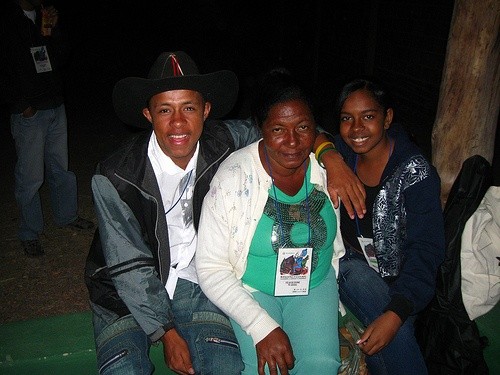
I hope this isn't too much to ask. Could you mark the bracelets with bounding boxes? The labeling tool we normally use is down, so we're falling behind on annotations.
[315,141,334,163]
[317,147,337,169]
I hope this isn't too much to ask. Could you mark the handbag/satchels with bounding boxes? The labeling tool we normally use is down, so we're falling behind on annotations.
[460,186,500,321]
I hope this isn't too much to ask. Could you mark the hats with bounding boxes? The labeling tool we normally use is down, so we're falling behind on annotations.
[112,51,239,129]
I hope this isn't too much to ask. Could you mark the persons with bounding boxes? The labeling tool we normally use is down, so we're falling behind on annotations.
[312,80,449,375]
[194,77,351,375]
[0,0,95,257]
[85,44,370,375]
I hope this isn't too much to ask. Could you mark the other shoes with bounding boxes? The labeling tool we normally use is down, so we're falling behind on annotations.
[22,238,47,258]
[56,216,94,232]
